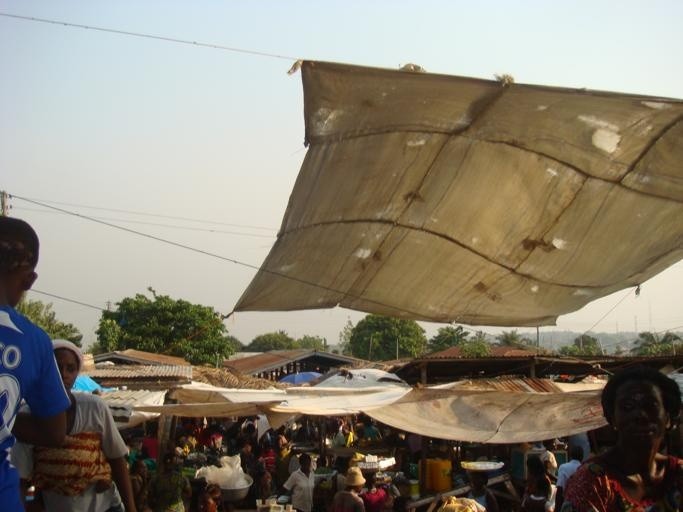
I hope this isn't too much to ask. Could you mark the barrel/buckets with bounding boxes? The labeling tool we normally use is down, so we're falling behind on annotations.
[408,477,419,500]
[419,458,452,491]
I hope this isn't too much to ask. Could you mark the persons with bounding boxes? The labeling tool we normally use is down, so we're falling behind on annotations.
[0,216,72,512]
[9,337,136,512]
[561,367,683,512]
[125,414,588,512]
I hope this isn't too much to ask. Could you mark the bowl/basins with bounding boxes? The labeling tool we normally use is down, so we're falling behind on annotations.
[218,473,253,500]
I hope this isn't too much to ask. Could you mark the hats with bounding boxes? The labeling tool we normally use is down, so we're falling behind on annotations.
[344,466,366,486]
[52,338,83,370]
